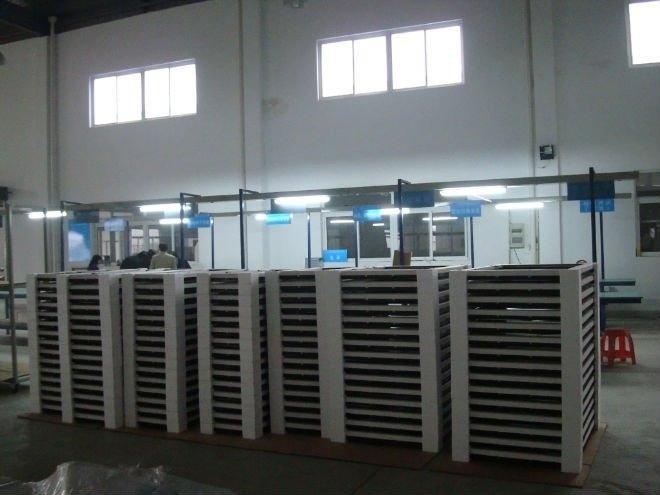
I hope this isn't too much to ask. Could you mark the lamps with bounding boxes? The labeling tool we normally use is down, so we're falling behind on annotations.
[140,203,190,213]
[440,185,507,197]
[28,211,68,219]
[422,216,458,222]
[330,220,355,224]
[274,195,330,206]
[539,145,554,160]
[495,202,545,211]
[158,218,188,226]
[372,223,384,227]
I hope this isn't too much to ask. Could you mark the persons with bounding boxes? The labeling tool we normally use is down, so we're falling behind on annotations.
[117,242,192,269]
[86,253,103,270]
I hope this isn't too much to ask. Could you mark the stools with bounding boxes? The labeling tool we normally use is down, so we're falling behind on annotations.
[601,328,635,369]
